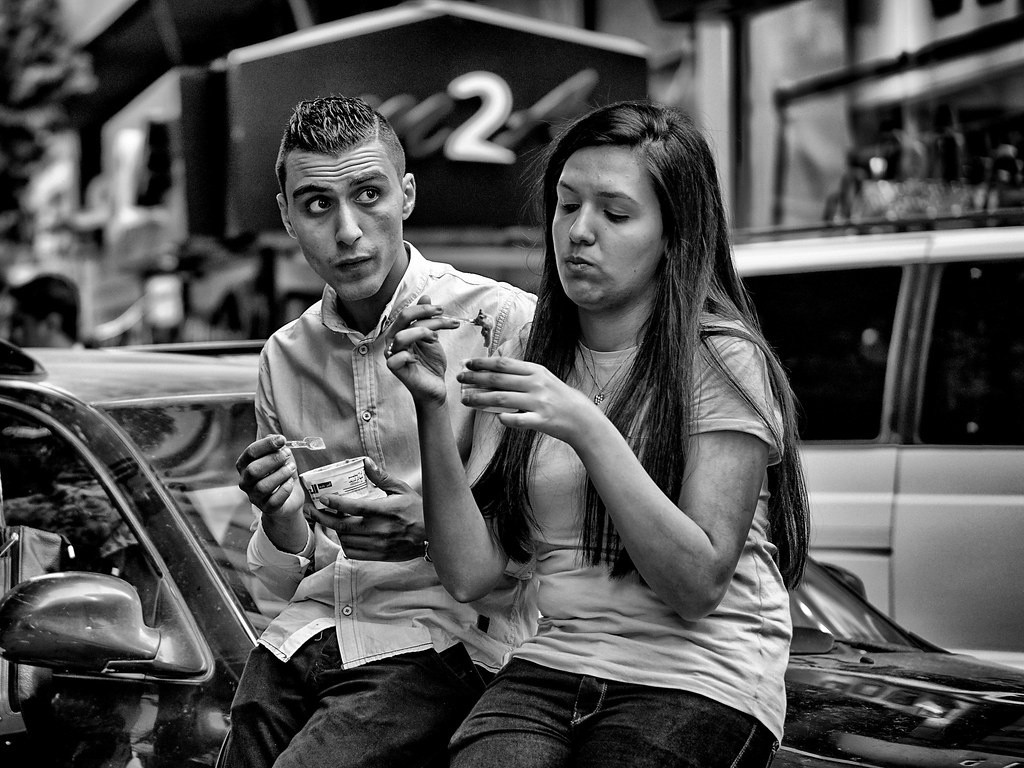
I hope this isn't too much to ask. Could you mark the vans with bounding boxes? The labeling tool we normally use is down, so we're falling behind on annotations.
[724,214,1024,657]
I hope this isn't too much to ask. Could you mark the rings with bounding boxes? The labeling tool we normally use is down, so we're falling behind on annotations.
[386,341,395,357]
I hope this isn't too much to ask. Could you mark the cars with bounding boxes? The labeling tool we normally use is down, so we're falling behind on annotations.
[0,340,1024,767]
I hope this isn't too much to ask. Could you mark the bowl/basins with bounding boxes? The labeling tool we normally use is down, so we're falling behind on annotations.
[299,455,369,509]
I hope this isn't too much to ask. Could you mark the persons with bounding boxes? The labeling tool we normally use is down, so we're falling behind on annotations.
[380,101,814,768]
[209,92,544,767]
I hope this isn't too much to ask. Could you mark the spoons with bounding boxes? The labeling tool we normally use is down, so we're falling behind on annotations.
[283,436,327,451]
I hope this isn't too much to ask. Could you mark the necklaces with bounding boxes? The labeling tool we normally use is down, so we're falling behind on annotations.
[573,339,642,407]
